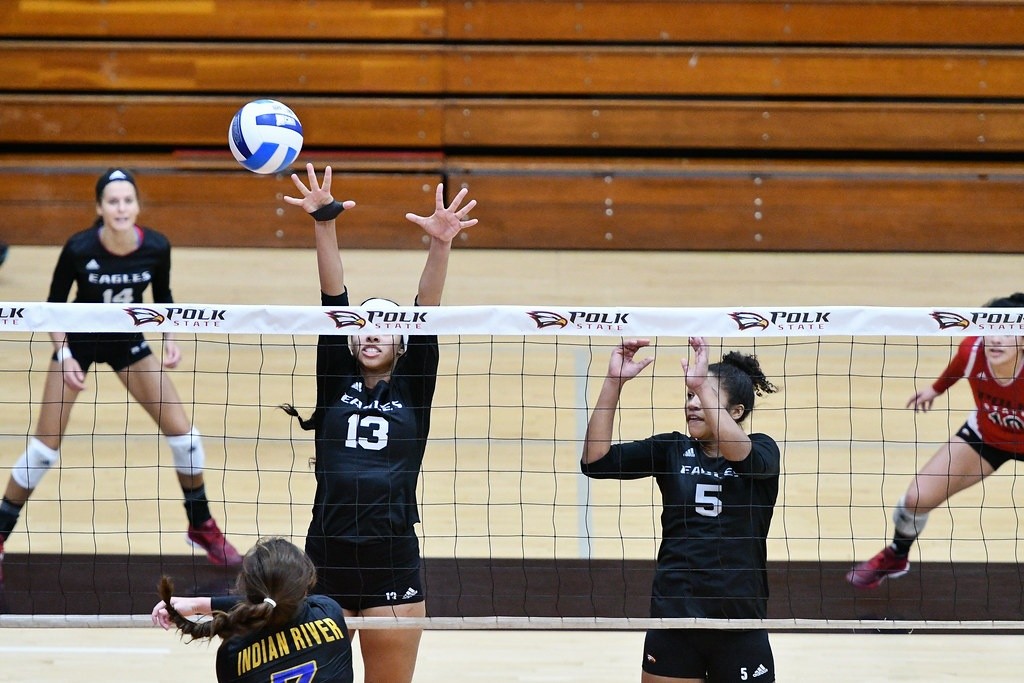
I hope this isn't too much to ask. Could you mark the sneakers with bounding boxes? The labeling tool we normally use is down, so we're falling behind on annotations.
[847,546,909,591]
[0,535,4,584]
[187,518,241,566]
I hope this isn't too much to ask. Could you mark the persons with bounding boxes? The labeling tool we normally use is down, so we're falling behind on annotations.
[0,168,241,567]
[151,538,353,683]
[276,162,479,683]
[847,293,1024,591]
[579,336,781,683]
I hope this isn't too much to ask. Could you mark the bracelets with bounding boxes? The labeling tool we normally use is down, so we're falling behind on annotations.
[57,347,73,364]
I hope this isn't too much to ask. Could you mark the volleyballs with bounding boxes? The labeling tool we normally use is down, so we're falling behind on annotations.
[227,99,307,174]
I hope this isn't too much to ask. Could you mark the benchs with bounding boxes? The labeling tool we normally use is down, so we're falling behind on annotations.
[0,151,1024,253]
[0,93,1024,150]
[0,0,1024,45]
[0,38,1024,100]
